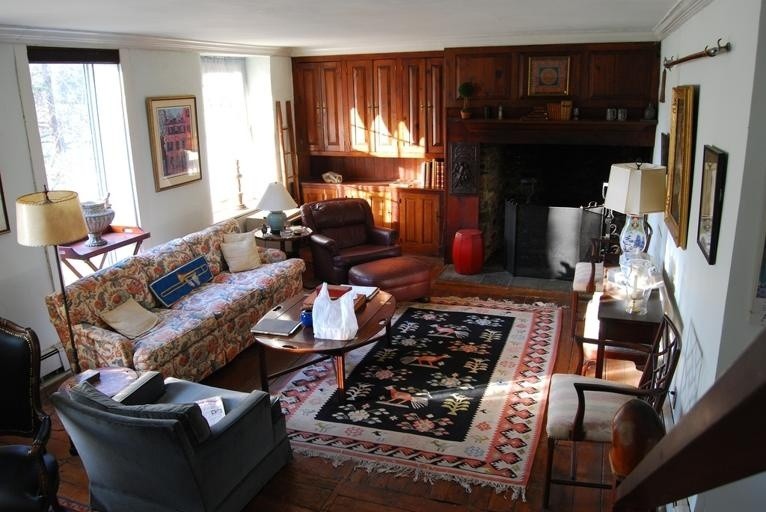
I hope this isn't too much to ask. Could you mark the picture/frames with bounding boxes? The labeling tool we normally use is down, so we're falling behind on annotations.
[696,144,727,264]
[663,85,700,249]
[146,94,202,192]
[527,55,571,96]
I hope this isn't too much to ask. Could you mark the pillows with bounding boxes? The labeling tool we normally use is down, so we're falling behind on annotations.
[100,297,159,338]
[220,230,263,274]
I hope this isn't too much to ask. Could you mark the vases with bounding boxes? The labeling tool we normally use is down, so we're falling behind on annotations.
[74,202,115,246]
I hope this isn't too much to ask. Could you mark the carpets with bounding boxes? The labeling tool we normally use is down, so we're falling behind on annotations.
[255,293,563,502]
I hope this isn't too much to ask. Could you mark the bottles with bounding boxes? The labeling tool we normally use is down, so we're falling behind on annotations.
[498,104,502,121]
[484,106,490,121]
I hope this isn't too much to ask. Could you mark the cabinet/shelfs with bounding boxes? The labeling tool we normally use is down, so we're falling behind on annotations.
[291,50,445,255]
[443,40,661,119]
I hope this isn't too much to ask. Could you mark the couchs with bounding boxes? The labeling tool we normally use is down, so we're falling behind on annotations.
[43,219,308,380]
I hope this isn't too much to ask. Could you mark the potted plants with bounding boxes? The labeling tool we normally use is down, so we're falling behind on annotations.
[459,82,475,109]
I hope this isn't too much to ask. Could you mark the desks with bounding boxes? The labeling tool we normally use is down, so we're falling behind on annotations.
[54,224,152,278]
[443,114,659,266]
[595,267,663,385]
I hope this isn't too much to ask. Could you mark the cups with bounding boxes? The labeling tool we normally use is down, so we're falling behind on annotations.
[573,107,579,120]
[618,109,627,121]
[607,109,617,120]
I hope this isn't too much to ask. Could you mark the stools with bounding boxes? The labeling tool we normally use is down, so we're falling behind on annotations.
[452,229,484,275]
[348,256,432,301]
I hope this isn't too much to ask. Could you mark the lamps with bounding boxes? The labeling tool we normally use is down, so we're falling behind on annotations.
[603,161,667,290]
[257,181,299,233]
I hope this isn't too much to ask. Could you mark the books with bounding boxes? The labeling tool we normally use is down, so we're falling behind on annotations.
[302,290,367,313]
[521,100,572,120]
[340,284,380,301]
[250,317,303,337]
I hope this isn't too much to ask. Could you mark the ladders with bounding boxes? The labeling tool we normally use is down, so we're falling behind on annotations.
[276,100,301,207]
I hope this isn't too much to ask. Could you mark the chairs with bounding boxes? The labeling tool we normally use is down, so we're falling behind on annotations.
[544,314,681,508]
[0,318,60,512]
[569,220,654,338]
[50,372,294,512]
[299,198,401,285]
